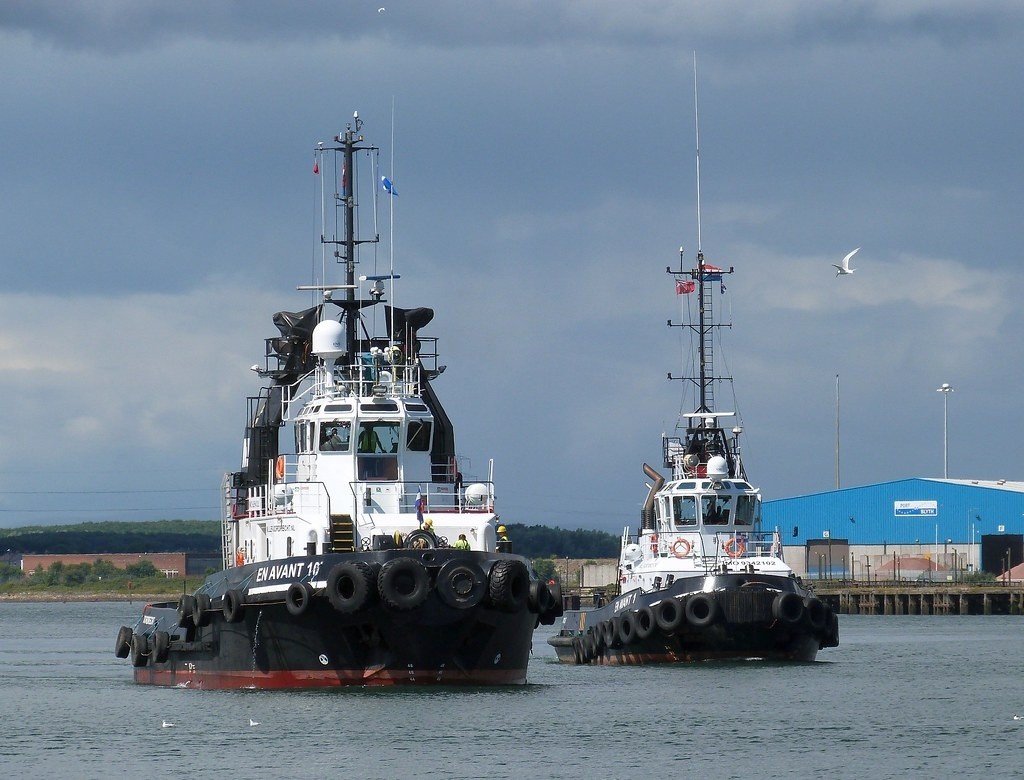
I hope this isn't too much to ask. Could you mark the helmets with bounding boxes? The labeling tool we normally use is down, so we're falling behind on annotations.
[497,526,507,532]
[425,519,433,526]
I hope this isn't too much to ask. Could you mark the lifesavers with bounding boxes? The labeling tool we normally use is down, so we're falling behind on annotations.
[130,635,148,668]
[377,557,432,613]
[684,593,717,627]
[275,457,284,480]
[325,561,374,615]
[603,615,620,649]
[671,539,690,558]
[489,559,531,614]
[192,593,212,627]
[632,605,654,639]
[403,529,439,548]
[772,590,805,622]
[176,594,197,629]
[653,596,681,631]
[617,610,636,645]
[115,626,133,660]
[802,596,828,631]
[724,539,743,557]
[286,581,313,616]
[571,621,605,664]
[531,580,563,629]
[151,631,170,663]
[223,589,246,621]
[820,610,840,648]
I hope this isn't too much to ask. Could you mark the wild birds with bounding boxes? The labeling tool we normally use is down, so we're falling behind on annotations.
[830,246,862,278]
[377,6,385,13]
[249,718,262,727]
[161,719,175,728]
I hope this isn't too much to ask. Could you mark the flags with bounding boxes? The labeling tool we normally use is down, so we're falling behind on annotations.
[381,176,399,198]
[701,264,721,282]
[342,155,348,187]
[413,484,424,523]
[675,277,696,294]
[720,284,727,295]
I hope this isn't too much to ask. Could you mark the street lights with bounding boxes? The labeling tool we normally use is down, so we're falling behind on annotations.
[936,382,954,478]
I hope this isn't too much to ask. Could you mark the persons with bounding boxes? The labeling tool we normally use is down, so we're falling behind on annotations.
[496,526,509,554]
[454,533,470,551]
[358,425,387,454]
[420,518,435,537]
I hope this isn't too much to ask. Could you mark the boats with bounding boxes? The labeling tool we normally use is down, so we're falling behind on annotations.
[546,47,839,666]
[114,97,541,692]
